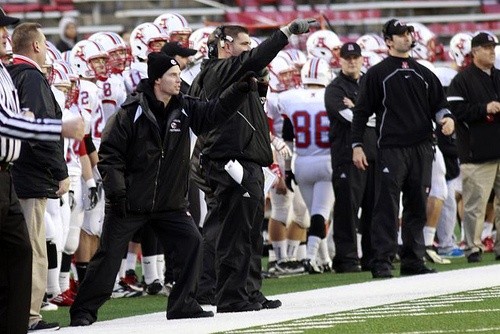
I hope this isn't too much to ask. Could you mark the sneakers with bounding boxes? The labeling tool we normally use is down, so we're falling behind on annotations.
[438,249,466,258]
[423,245,452,264]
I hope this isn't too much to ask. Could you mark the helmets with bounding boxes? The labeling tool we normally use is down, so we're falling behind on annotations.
[1,14,475,89]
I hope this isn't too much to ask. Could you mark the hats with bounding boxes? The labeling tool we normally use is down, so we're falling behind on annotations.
[161,41,196,56]
[382,19,415,41]
[0,9,20,27]
[147,51,180,82]
[340,42,362,57]
[472,32,500,47]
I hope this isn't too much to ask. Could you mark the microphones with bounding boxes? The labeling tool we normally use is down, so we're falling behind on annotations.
[411,41,416,48]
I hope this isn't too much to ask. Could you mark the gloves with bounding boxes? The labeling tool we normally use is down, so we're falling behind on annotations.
[233,70,257,92]
[272,136,292,159]
[282,170,298,192]
[88,186,99,210]
[289,19,317,34]
[110,193,131,220]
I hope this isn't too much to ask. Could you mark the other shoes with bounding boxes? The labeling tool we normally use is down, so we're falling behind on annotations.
[460,237,494,253]
[168,308,213,319]
[373,269,393,278]
[72,318,91,326]
[112,269,162,298]
[28,320,60,332]
[261,259,332,279]
[217,293,282,313]
[401,265,436,276]
[468,252,482,264]
[40,277,76,311]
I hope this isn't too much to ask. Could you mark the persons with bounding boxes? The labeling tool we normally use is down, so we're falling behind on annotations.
[152,13,342,313]
[325,42,397,273]
[398,23,473,264]
[350,18,455,279]
[448,31,500,263]
[0,9,167,334]
[69,52,258,326]
